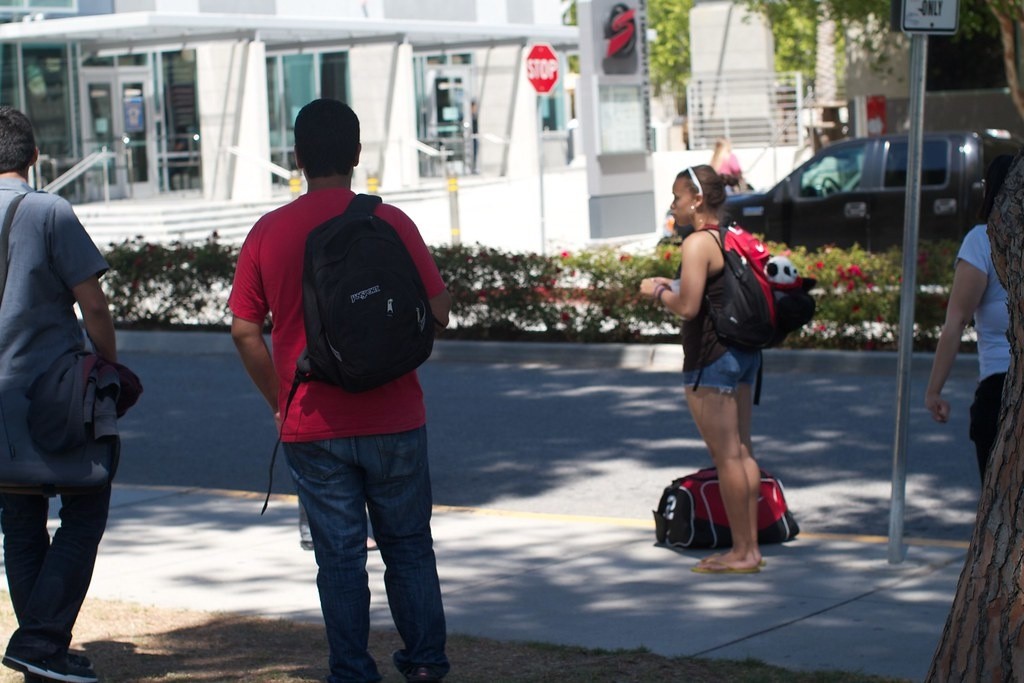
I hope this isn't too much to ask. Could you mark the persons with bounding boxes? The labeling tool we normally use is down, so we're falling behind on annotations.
[925,154,1013,488]
[711,137,747,195]
[226,98,451,683]
[0,108,122,683]
[641,164,767,571]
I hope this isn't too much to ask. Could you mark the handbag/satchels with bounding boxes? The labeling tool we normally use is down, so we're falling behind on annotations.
[0,376,117,494]
[653,462,800,549]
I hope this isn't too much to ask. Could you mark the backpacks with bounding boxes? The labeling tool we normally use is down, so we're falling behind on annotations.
[692,216,818,350]
[296,194,435,392]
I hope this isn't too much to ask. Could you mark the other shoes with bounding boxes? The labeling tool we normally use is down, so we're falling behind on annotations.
[406,665,441,683]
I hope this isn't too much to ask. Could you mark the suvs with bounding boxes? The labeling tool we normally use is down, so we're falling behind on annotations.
[665,129,1023,252]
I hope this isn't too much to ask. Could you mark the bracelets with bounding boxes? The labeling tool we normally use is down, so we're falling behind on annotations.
[658,288,665,299]
[653,285,660,298]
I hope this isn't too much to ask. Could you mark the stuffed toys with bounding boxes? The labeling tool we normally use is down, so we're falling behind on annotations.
[764,256,798,299]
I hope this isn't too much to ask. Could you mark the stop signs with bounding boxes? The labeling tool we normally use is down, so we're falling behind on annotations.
[526,42,560,95]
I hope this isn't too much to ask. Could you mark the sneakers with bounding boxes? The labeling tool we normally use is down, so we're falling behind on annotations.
[24,653,94,683]
[2,651,99,683]
